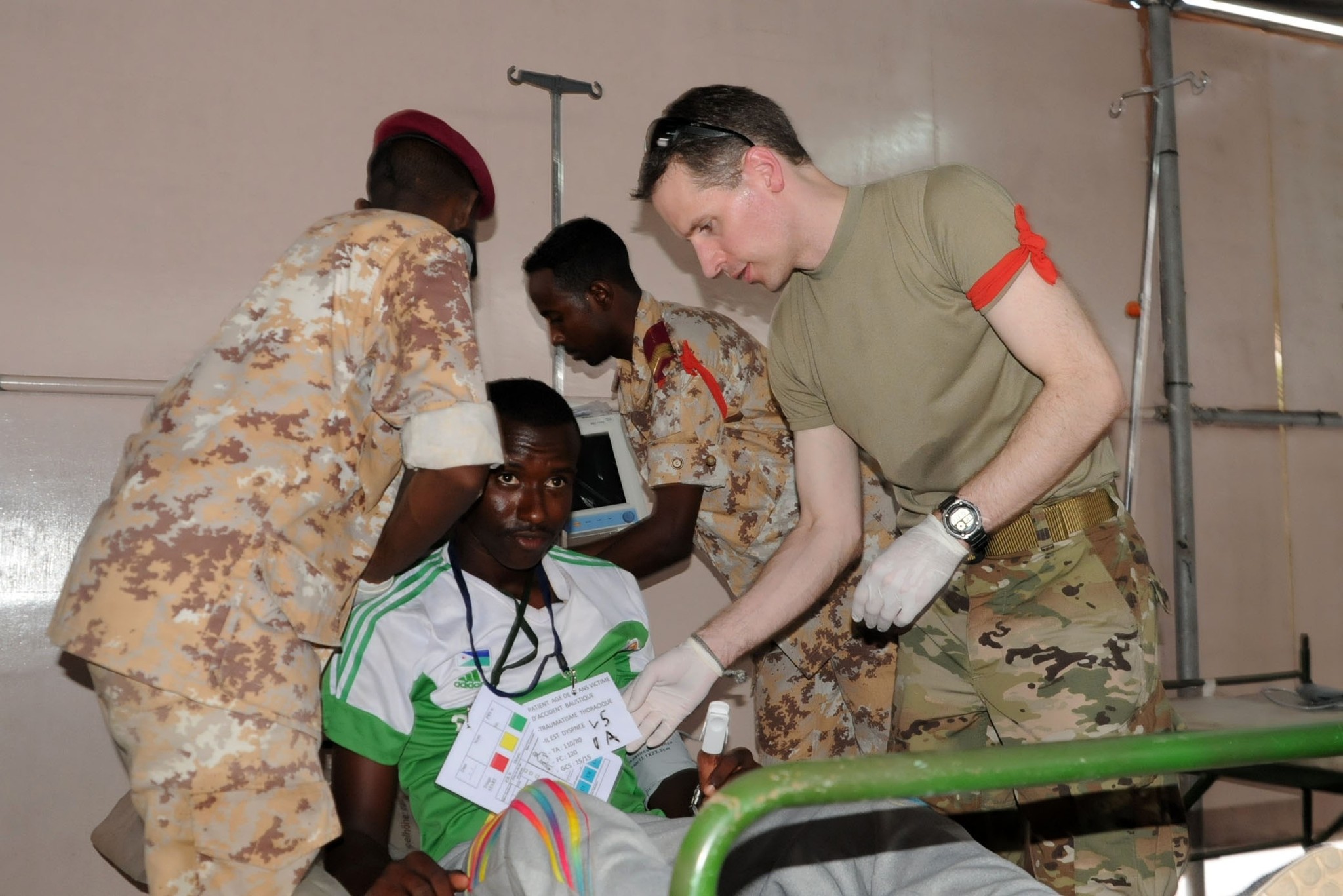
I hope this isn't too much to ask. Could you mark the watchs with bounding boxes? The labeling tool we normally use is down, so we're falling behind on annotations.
[937,495,988,562]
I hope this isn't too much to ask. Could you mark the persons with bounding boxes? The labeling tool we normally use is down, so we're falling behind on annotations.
[520,213,895,765]
[607,88,1192,896]
[315,378,1343,896]
[42,107,498,896]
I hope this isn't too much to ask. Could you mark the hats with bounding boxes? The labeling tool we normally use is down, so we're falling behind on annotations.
[374,109,495,221]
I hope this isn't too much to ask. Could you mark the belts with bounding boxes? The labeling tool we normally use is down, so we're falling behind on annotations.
[961,478,1120,564]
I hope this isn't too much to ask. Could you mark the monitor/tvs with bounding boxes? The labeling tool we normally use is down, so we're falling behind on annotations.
[570,432,627,512]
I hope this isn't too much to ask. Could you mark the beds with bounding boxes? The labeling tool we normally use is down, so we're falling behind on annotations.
[90,712,1343,895]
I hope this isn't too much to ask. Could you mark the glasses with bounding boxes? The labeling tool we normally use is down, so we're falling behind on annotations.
[645,118,754,157]
